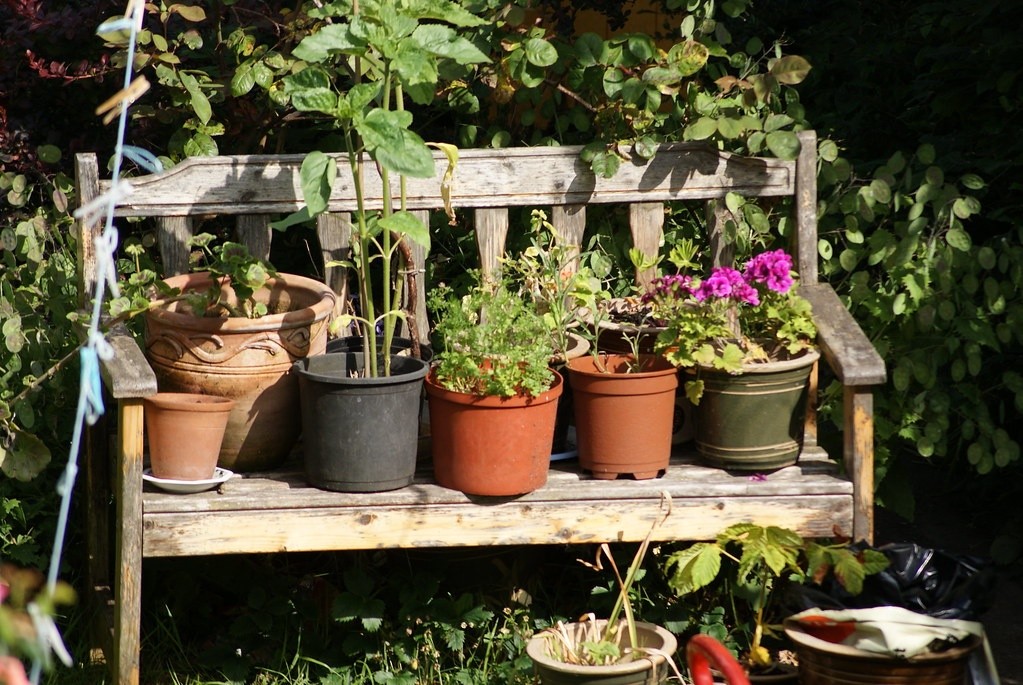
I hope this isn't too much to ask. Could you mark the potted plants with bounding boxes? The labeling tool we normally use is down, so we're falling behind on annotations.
[143,263,819,497]
[524,491,989,685]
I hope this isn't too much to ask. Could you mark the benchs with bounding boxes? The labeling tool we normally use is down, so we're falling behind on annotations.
[68,125,887,685]
